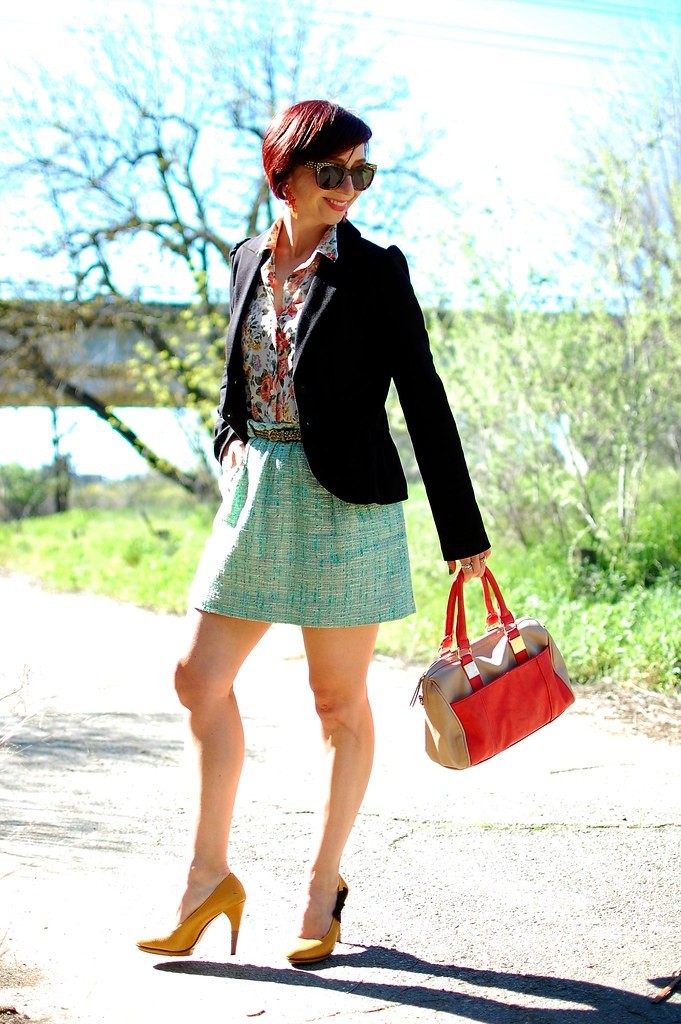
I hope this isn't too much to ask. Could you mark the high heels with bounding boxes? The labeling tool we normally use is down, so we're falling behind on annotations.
[285,874,348,964]
[135,872,246,956]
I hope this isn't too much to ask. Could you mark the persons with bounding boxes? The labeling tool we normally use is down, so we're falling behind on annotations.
[136,100,491,964]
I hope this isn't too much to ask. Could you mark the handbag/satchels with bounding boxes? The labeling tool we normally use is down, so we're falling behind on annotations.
[409,565,575,770]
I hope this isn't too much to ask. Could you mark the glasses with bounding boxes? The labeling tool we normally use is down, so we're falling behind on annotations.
[302,160,377,191]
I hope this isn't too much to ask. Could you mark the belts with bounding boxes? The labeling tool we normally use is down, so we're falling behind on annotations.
[254,428,302,442]
